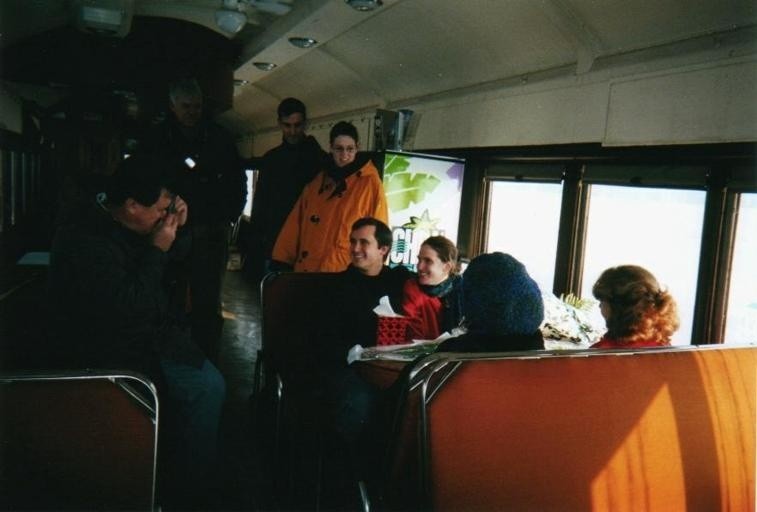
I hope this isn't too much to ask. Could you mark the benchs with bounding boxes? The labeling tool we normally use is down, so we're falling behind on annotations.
[3,368,156,511]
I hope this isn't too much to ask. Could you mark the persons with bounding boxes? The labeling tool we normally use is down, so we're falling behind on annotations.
[147,76,247,365]
[271,120,389,273]
[249,97,328,273]
[397,235,464,509]
[584,263,681,350]
[314,215,413,511]
[41,154,227,511]
[433,249,545,353]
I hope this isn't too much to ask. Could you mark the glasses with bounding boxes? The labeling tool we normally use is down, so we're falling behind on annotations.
[331,144,357,155]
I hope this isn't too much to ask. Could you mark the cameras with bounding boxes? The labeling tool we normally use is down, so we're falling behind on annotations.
[167,197,179,218]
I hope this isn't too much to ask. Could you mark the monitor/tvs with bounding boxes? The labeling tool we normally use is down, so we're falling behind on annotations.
[371,108,403,148]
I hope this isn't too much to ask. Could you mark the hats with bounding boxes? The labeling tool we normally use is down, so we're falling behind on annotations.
[461,251,541,338]
